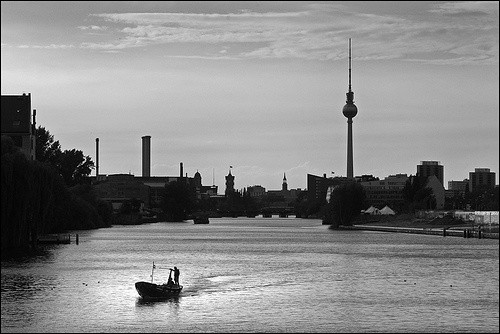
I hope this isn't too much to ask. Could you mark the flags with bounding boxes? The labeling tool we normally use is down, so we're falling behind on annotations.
[332,171,335,174]
[230,165,233,168]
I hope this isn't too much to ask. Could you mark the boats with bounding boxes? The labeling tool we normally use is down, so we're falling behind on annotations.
[134,260,183,297]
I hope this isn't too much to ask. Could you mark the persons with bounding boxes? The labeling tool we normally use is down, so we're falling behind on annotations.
[170,266,180,287]
